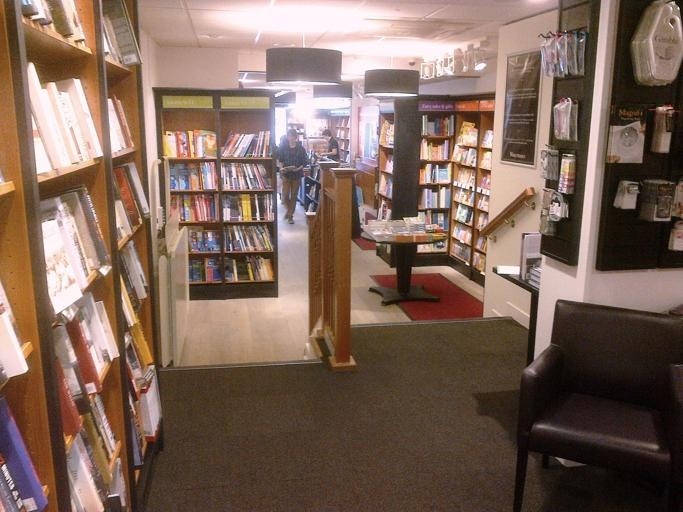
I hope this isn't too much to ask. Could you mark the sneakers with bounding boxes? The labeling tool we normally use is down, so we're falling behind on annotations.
[285,211,294,225]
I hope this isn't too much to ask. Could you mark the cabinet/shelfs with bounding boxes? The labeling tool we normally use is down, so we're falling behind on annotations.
[151,87,280,300]
[374,94,494,286]
[0,0,163,510]
[286,110,352,215]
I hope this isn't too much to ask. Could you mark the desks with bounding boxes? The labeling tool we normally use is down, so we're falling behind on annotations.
[492,264,538,367]
[360,226,448,304]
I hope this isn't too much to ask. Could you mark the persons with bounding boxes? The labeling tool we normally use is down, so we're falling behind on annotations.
[277,136,287,199]
[278,128,308,224]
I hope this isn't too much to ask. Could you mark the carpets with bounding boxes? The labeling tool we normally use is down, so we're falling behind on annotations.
[144,317,681,511]
[370,273,483,322]
[354,236,375,250]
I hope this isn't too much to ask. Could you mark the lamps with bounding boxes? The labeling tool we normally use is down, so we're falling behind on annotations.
[265,24,420,108]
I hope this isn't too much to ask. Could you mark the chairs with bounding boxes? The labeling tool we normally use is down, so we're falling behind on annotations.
[511,299,682,512]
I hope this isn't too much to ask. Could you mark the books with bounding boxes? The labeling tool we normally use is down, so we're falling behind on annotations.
[162,129,276,287]
[530,268,540,277]
[527,273,540,282]
[526,280,541,288]
[1,1,165,512]
[289,111,491,282]
